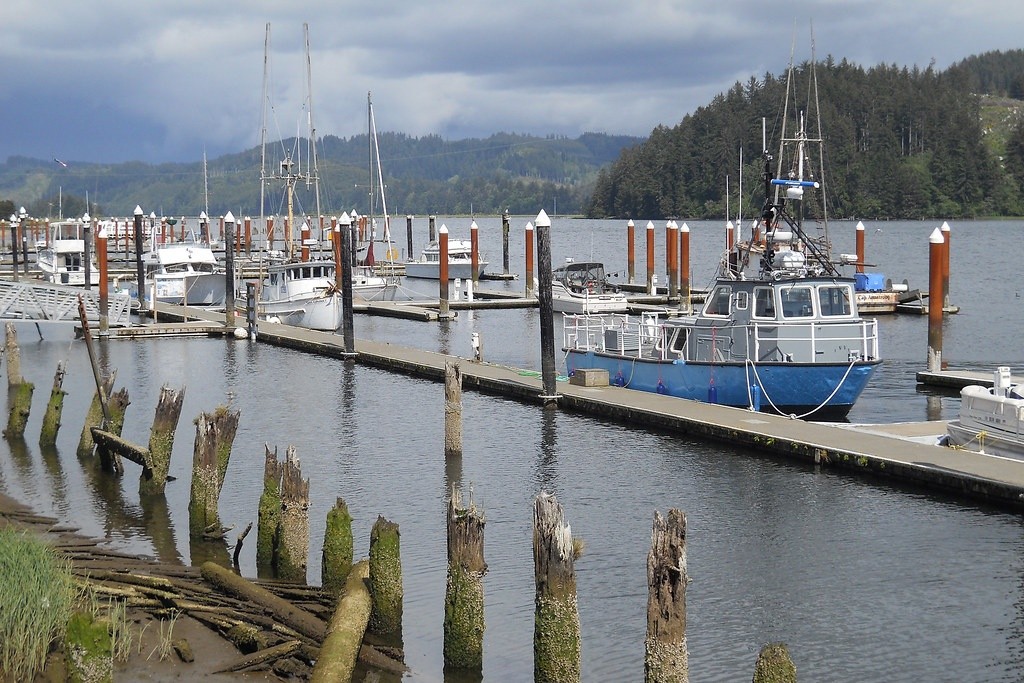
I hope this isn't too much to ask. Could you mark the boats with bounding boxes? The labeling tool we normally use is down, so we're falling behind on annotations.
[562,148,884,422]
[103,221,168,236]
[403,238,490,281]
[34,221,100,286]
[130,225,226,308]
[234,232,256,249]
[534,261,630,315]
[946,365,1024,460]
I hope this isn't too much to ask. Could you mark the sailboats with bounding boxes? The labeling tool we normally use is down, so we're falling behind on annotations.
[233,20,344,332]
[718,15,878,302]
[352,90,400,300]
[195,151,218,249]
